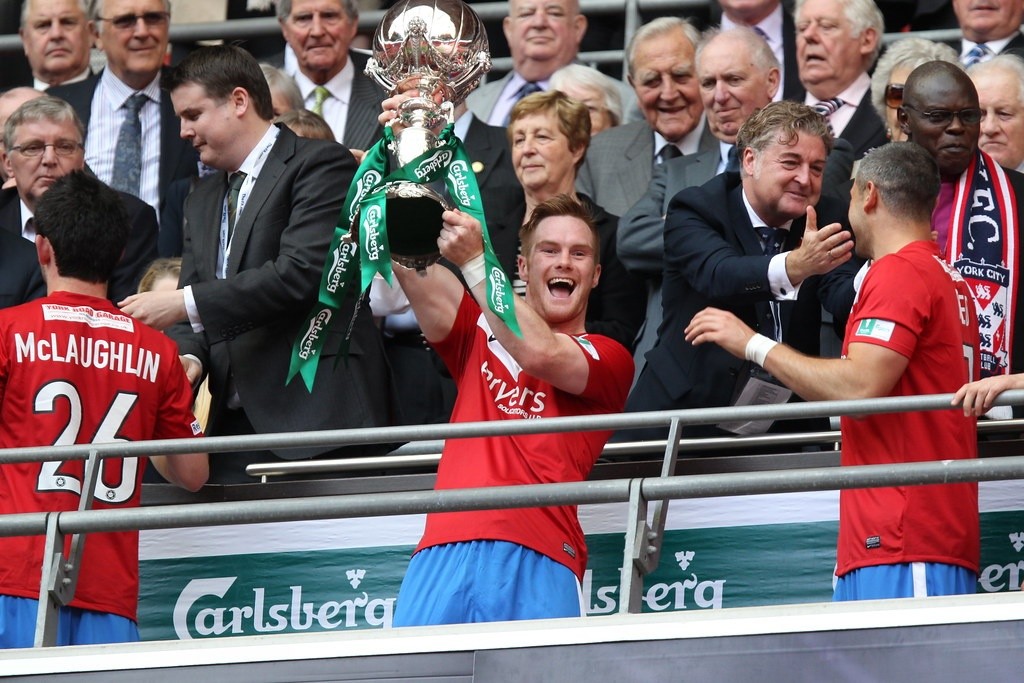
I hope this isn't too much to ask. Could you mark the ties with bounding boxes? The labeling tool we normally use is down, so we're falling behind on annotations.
[656,144,684,163]
[961,44,989,70]
[310,86,330,118]
[225,171,247,254]
[724,145,742,172]
[499,82,544,130]
[810,98,846,139]
[109,94,150,198]
[752,226,789,342]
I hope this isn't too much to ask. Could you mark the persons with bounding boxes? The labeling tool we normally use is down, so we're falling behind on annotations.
[687,142,981,599]
[0,0,1024,483]
[951,372,1024,417]
[379,94,635,628]
[0,169,209,649]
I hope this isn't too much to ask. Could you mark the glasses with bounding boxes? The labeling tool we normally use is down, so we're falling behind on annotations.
[883,83,906,110]
[94,12,168,29]
[901,103,982,125]
[10,140,86,157]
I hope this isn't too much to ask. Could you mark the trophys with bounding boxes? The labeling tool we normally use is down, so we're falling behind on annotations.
[341,0,493,277]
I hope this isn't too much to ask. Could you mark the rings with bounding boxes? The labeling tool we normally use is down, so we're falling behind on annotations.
[828,250,835,259]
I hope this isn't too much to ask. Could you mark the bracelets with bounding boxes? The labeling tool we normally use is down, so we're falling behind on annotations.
[460,253,486,289]
[746,332,777,368]
